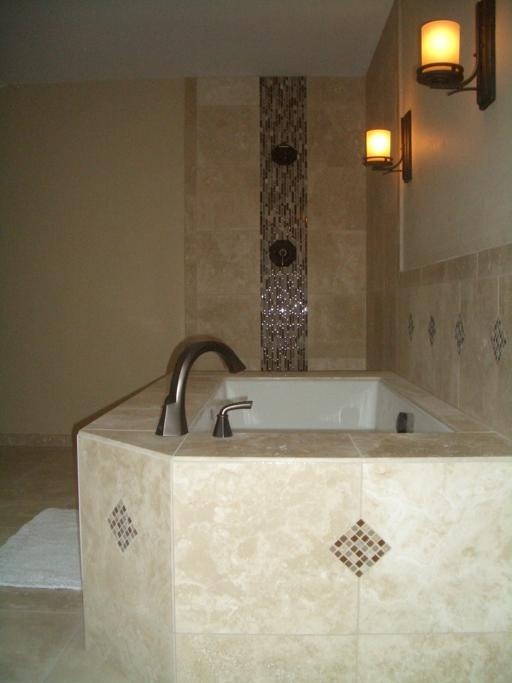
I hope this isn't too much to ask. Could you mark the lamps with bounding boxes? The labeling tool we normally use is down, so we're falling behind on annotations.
[363,106,414,185]
[415,0,499,111]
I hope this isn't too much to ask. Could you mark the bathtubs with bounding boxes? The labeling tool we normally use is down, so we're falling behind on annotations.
[185,374,450,434]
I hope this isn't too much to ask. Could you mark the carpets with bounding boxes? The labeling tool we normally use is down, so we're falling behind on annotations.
[0,505,83,591]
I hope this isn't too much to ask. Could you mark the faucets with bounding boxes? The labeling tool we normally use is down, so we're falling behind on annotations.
[213,401,253,438]
[154,340,248,437]
